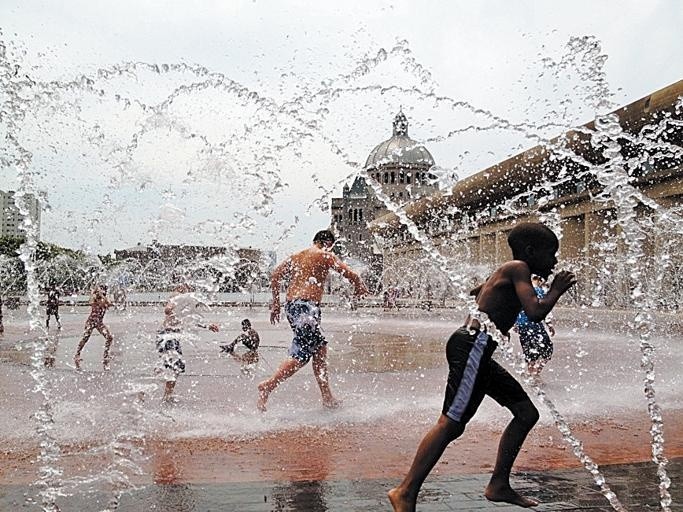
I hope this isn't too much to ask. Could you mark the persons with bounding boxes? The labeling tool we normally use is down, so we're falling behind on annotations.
[39,281,62,329]
[155,284,219,403]
[73,283,113,372]
[386,222,578,512]
[513,275,555,387]
[218,318,260,352]
[258,229,367,409]
